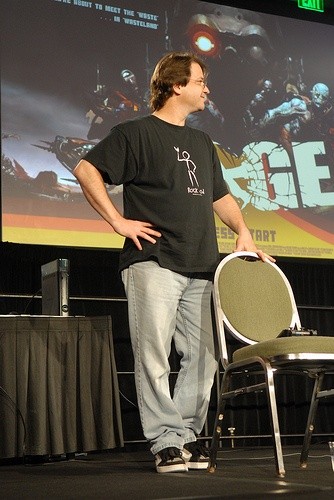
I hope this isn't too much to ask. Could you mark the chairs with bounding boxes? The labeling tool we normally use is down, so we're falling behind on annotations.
[204,252,334,478]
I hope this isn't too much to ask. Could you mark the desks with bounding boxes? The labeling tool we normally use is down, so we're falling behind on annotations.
[0,312,123,462]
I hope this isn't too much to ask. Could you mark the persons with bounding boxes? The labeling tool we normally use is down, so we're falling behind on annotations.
[75,51,279,476]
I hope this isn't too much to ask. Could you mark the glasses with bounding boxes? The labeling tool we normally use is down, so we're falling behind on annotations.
[181,75,208,88]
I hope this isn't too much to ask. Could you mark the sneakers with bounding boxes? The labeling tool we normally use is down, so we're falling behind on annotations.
[153,447,189,473]
[180,441,217,470]
